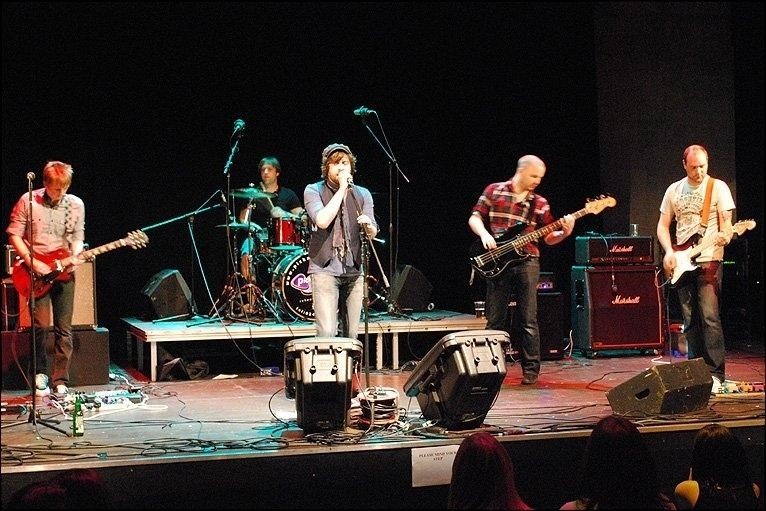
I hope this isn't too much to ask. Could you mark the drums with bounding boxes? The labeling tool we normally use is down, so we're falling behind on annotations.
[268,217,303,246]
[257,239,272,254]
[271,250,314,322]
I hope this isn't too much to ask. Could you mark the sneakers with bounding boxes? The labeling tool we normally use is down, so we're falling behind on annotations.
[710,375,721,394]
[36,373,50,397]
[56,385,68,393]
[521,373,538,384]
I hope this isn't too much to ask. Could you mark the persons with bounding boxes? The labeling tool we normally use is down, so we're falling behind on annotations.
[559,413,677,511]
[447,432,536,511]
[302,142,380,340]
[238,156,303,316]
[468,153,576,385]
[656,144,737,397]
[6,159,96,399]
[674,423,762,511]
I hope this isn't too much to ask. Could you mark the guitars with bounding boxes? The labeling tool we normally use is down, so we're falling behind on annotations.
[664,219,756,289]
[11,231,150,298]
[469,192,617,278]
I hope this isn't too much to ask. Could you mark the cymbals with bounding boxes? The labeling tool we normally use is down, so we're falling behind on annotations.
[215,223,248,229]
[230,188,275,198]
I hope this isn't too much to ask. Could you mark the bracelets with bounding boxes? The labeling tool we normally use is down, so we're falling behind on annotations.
[21,253,30,261]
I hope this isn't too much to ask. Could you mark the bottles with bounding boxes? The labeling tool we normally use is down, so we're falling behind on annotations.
[72,397,84,436]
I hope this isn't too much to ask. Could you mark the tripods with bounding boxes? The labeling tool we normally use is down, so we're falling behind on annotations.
[0,201,72,437]
[358,116,421,322]
[186,135,285,327]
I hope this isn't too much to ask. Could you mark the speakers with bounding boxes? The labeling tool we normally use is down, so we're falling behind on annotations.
[575,237,653,263]
[604,357,713,418]
[517,292,563,360]
[571,266,662,358]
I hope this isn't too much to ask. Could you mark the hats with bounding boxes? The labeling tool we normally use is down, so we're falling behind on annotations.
[322,143,351,160]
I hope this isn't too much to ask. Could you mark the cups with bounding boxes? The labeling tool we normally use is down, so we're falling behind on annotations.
[629,224,639,237]
[474,301,486,319]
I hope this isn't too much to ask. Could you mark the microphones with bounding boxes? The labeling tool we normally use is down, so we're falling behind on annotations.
[354,107,370,116]
[338,169,355,187]
[26,172,35,188]
[234,119,246,131]
